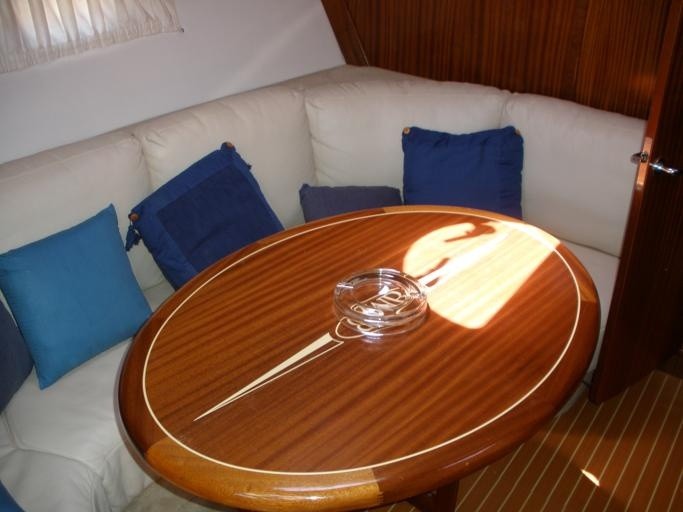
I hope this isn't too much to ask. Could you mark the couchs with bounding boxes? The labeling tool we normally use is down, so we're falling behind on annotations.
[0,64,647,511]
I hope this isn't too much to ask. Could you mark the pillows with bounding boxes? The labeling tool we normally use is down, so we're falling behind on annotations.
[0,205,153,410]
[299,184,401,222]
[401,126,523,218]
[125,141,284,291]
